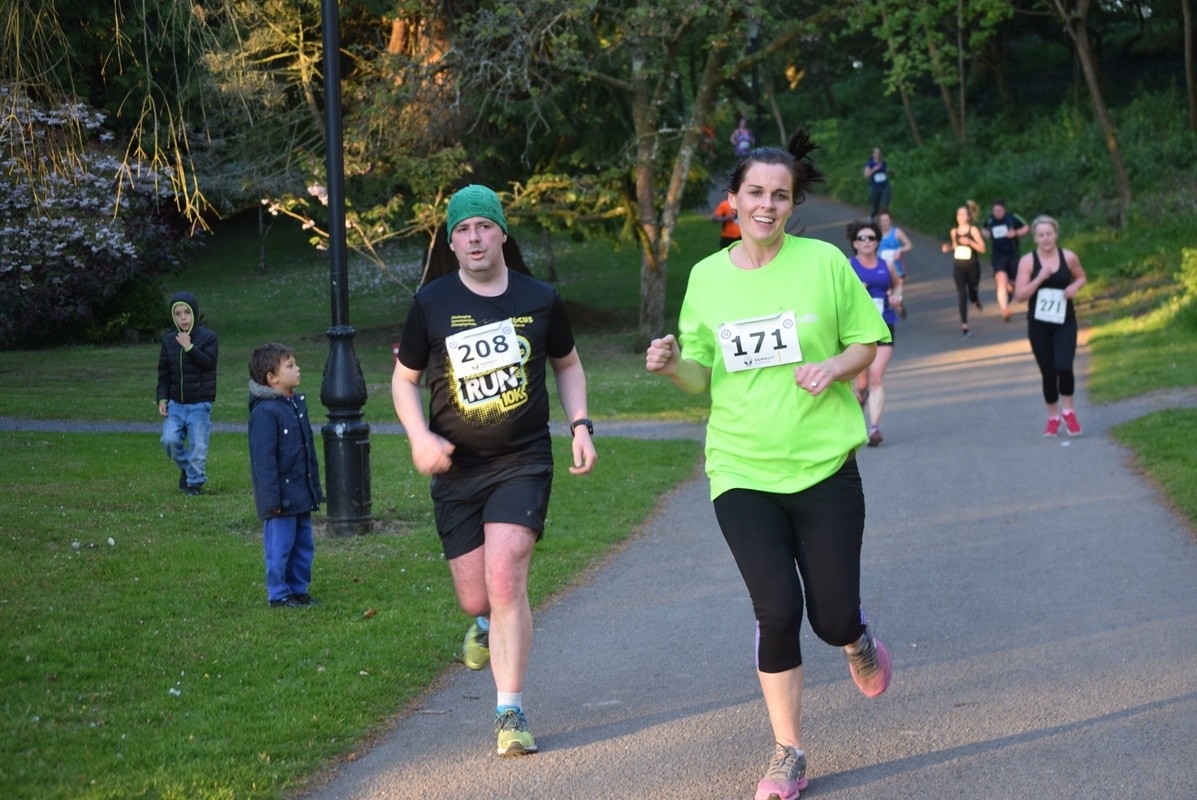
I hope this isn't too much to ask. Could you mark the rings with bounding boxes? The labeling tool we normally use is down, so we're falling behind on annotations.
[811,381,818,388]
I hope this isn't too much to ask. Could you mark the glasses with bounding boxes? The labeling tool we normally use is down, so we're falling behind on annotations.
[854,235,878,241]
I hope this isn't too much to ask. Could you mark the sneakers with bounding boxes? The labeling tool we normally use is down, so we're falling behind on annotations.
[463,616,490,670]
[1008,285,1015,301]
[867,428,883,447]
[755,741,807,800]
[961,329,972,340]
[494,704,537,755]
[1060,409,1083,436]
[1002,310,1013,321]
[974,302,983,313]
[857,390,869,408]
[842,608,892,700]
[1043,418,1061,435]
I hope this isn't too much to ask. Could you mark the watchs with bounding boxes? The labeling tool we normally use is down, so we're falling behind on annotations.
[569,418,594,435]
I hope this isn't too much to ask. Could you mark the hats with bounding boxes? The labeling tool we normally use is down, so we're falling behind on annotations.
[445,184,508,243]
[721,184,735,194]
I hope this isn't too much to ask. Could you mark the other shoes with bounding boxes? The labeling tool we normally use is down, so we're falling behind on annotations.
[178,469,187,491]
[293,593,319,605]
[186,487,200,496]
[269,595,305,608]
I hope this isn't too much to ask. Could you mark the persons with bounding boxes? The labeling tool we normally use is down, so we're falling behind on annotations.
[155,291,218,497]
[248,340,323,608]
[862,148,896,223]
[644,146,891,800]
[875,212,915,318]
[698,122,718,155]
[392,184,597,755]
[712,199,741,249]
[847,219,903,448]
[730,117,755,162]
[940,199,1030,335]
[1014,214,1087,437]
